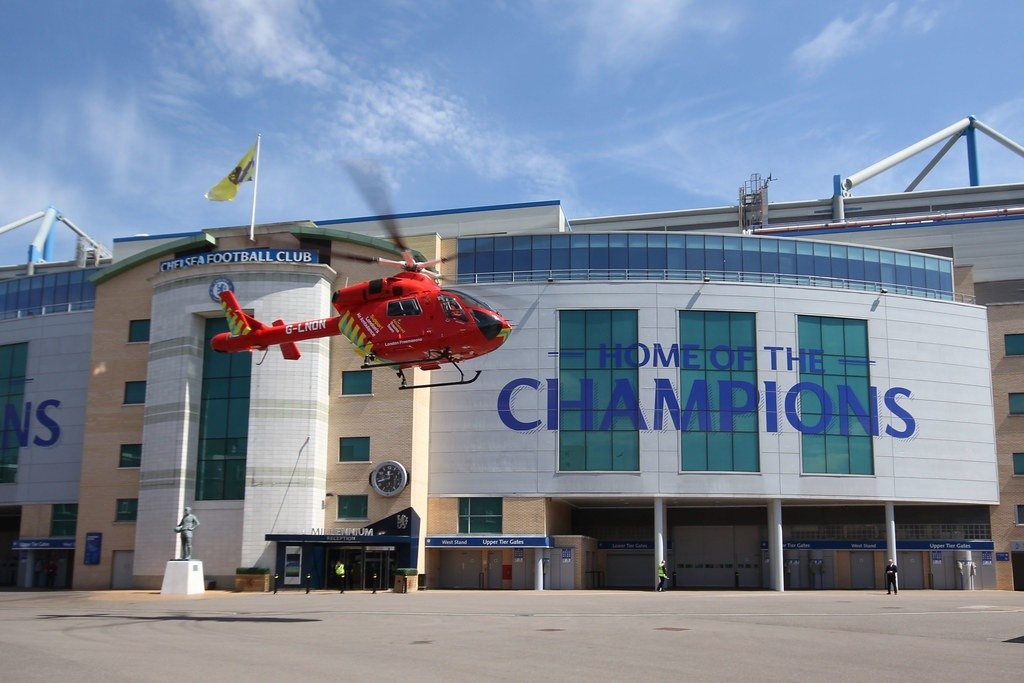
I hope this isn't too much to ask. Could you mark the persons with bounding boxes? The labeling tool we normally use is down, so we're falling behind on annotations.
[174,507,201,559]
[333,561,352,593]
[447,300,465,319]
[657,560,668,592]
[885,559,898,595]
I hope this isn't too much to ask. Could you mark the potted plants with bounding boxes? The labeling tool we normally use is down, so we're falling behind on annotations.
[235,567,271,593]
[395,568,419,593]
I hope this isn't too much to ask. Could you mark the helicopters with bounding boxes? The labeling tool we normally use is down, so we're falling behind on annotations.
[209,145,540,391]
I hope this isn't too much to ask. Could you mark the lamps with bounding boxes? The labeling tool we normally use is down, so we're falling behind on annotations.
[704,277,710,282]
[881,289,887,294]
[548,279,554,283]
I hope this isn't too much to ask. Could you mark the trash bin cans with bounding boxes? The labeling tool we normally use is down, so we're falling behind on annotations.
[236,567,270,591]
[394,568,418,592]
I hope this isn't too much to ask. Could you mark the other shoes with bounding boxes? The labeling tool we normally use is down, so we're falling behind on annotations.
[887,592,891,594]
[895,592,897,594]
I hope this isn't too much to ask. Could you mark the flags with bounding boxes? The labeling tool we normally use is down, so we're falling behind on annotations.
[204,139,259,201]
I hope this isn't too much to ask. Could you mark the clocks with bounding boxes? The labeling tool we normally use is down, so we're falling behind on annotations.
[372,459,409,499]
